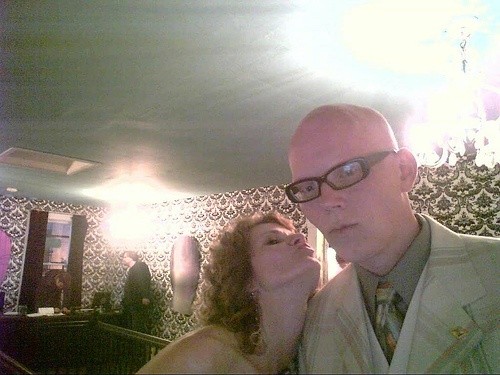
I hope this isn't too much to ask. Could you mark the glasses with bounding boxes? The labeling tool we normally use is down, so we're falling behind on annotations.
[285,150,396,204]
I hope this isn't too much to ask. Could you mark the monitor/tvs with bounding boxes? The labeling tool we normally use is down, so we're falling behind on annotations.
[92,292,111,311]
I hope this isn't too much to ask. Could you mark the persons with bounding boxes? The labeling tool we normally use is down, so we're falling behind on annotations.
[121,250,152,372]
[287,102,500,375]
[32,268,72,316]
[135,210,322,375]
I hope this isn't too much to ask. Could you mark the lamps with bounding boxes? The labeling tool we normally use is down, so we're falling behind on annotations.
[405,11,500,168]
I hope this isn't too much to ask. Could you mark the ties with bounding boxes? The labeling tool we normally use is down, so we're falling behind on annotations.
[372,281,405,365]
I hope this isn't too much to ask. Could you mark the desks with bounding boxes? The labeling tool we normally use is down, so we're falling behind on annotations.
[0,306,132,369]
